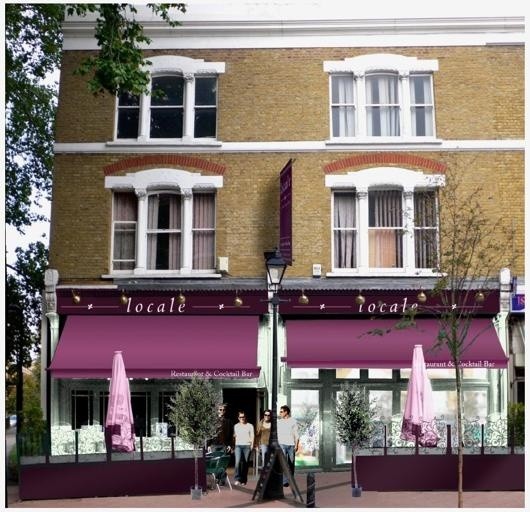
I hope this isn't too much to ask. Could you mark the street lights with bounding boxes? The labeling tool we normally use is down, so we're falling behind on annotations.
[261,246,289,502]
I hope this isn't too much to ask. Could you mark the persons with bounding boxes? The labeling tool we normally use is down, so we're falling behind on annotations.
[256,408,272,469]
[231,410,254,489]
[277,405,301,487]
[207,404,232,489]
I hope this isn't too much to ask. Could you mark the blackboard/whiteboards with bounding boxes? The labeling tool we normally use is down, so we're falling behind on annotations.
[255,443,279,495]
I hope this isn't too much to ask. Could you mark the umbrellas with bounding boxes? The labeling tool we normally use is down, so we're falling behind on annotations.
[104,350,137,452]
[398,343,441,448]
